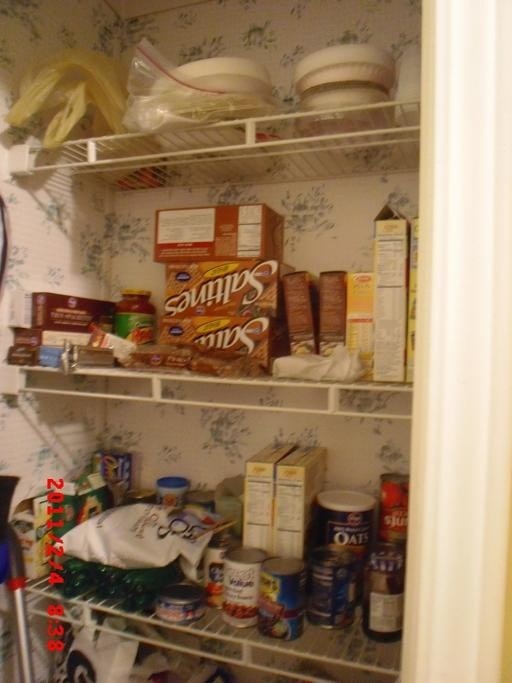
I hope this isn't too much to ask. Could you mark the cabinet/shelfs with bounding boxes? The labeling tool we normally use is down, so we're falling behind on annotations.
[4,98,420,682]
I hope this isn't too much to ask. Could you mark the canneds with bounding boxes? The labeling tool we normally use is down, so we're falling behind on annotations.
[362,550,405,643]
[258,556,308,641]
[306,545,357,631]
[222,546,268,629]
[155,582,206,624]
[203,537,233,610]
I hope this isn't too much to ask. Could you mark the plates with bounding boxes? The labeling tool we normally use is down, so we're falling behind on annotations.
[154,44,399,134]
